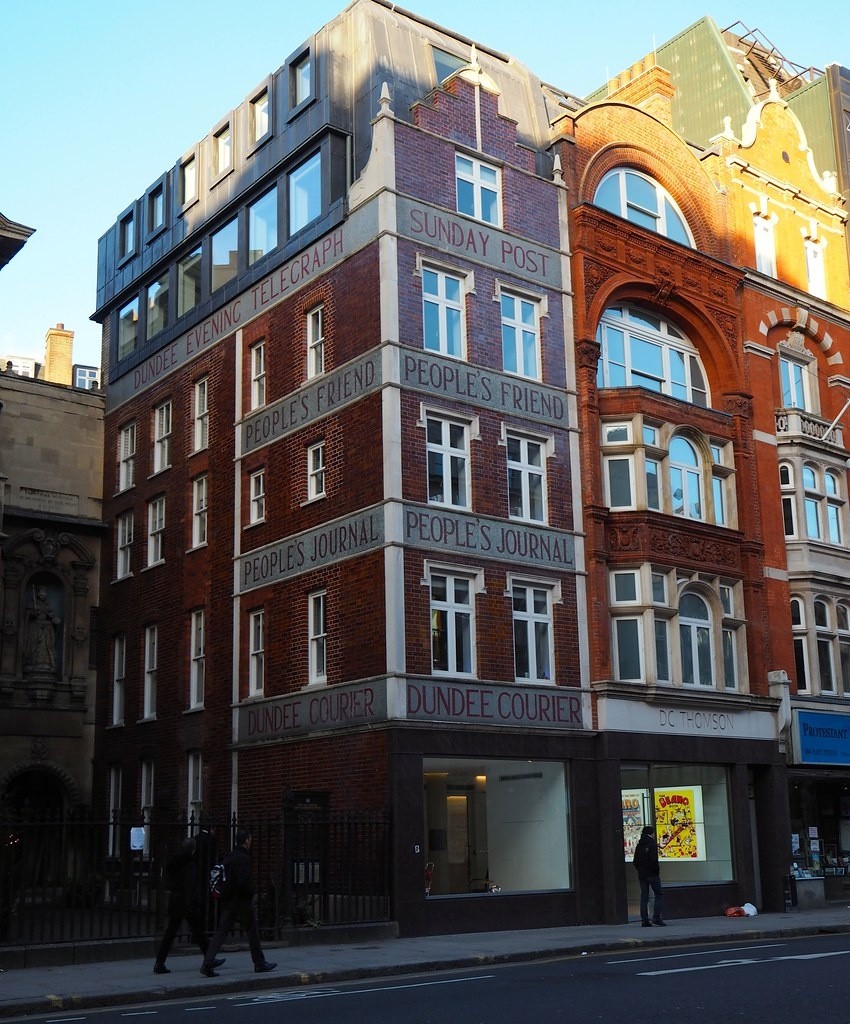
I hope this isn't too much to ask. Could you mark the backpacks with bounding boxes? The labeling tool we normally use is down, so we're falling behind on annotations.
[210,856,239,897]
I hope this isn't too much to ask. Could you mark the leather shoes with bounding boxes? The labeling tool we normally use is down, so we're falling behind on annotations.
[255,962,277,973]
[154,963,172,973]
[213,959,225,967]
[201,966,220,977]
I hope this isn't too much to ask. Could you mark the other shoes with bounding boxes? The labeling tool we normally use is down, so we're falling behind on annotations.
[652,917,666,926]
[642,920,653,927]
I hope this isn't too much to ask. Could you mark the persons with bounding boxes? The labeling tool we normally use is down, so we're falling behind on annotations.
[633,826,667,927]
[152,820,278,978]
